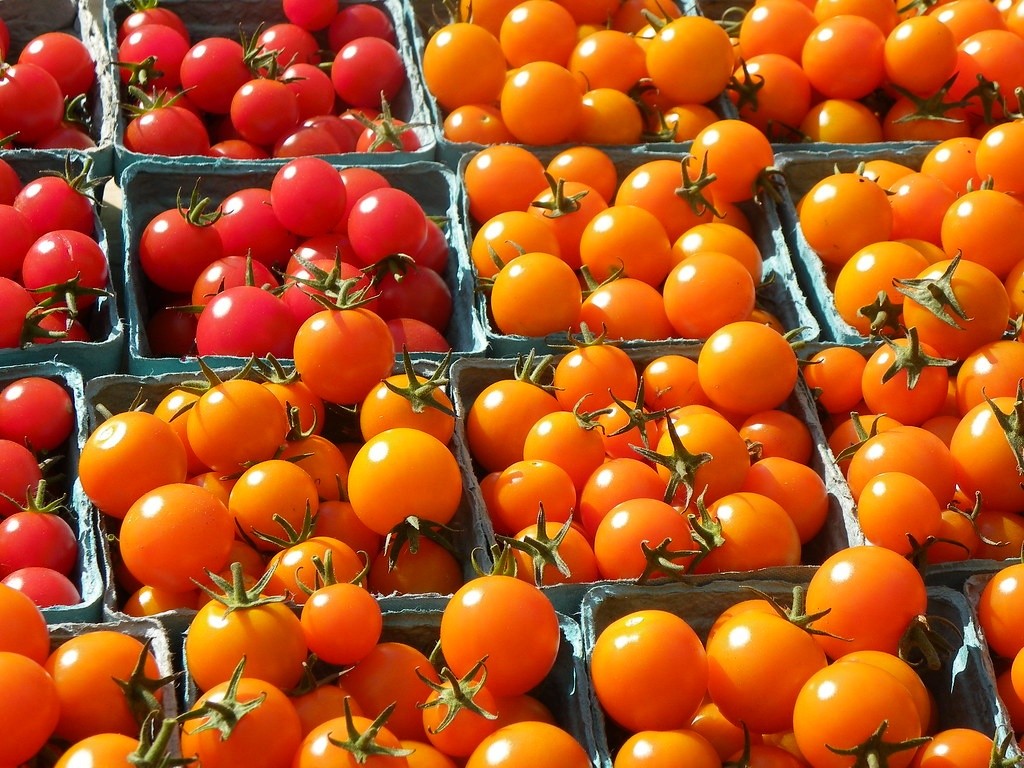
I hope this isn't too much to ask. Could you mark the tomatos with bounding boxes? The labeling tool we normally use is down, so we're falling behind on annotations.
[0,0,1024,768]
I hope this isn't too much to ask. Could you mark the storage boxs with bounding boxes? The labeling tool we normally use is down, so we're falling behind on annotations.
[85,359,495,650]
[0,0,115,183]
[405,0,732,174]
[450,343,869,612]
[182,610,602,768]
[456,148,822,355]
[120,160,491,377]
[0,360,105,624]
[580,580,1020,768]
[767,145,937,343]
[103,0,436,183]
[0,147,125,381]
[46,617,181,768]
